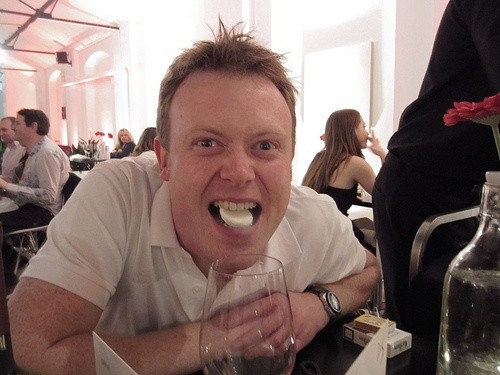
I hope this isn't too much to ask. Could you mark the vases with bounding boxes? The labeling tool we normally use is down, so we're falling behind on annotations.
[437,171,500,374]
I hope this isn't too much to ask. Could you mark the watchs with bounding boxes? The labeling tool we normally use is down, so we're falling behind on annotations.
[1,183,8,194]
[302,283,344,325]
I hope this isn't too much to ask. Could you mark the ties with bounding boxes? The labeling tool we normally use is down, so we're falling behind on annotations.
[12,151,29,184]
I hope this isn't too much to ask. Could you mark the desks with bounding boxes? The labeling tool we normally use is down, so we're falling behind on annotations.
[194,319,436,374]
[70,159,107,173]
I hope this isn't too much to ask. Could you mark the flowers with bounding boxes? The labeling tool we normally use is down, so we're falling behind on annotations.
[444,92,500,162]
[78,131,113,157]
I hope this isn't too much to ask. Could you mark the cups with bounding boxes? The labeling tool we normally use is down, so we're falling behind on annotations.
[199,254,296,375]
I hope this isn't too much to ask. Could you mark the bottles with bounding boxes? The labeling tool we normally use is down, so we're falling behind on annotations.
[436,172,500,375]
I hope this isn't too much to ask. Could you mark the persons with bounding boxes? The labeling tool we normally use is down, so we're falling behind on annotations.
[129,126,158,156]
[109,128,137,159]
[8,19,383,375]
[372,0,500,375]
[1,106,72,235]
[301,109,378,258]
[0,116,28,183]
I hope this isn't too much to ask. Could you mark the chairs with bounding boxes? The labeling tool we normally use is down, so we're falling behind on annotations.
[4,226,49,276]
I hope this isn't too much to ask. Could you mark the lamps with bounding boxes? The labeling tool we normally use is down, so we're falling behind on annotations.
[0,0,119,71]
